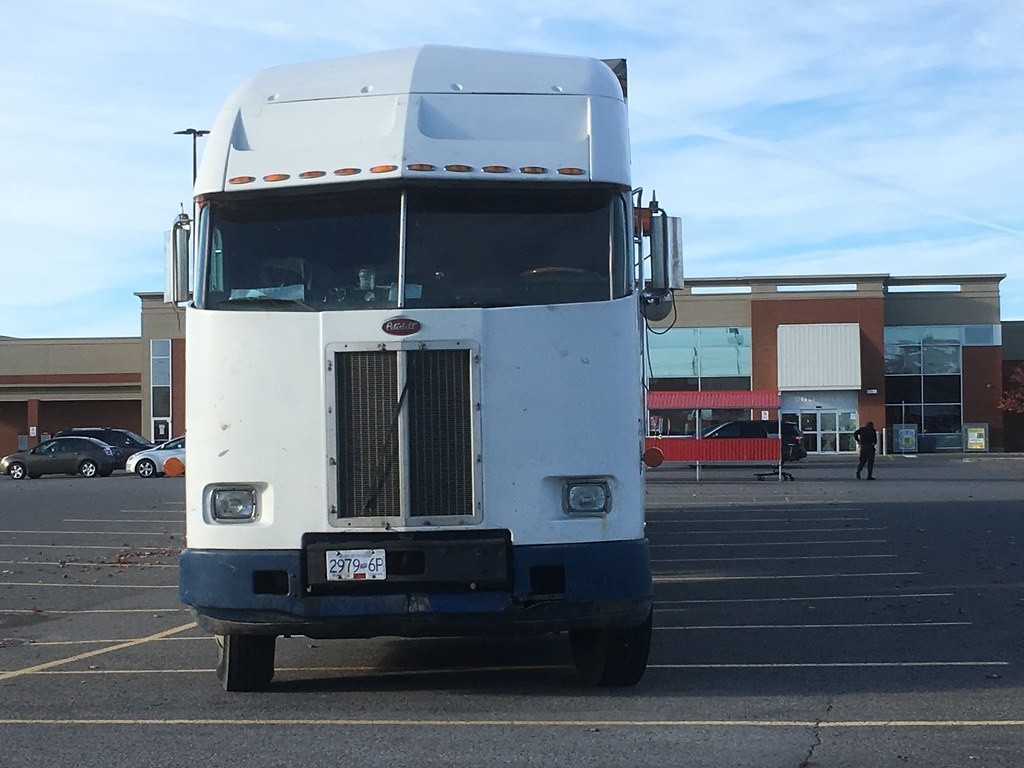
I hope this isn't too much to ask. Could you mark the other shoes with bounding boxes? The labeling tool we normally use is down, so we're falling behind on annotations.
[856,471,860,479]
[866,476,875,480]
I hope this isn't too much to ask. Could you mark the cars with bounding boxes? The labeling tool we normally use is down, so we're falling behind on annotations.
[52,429,160,472]
[125,435,186,478]
[0,436,121,478]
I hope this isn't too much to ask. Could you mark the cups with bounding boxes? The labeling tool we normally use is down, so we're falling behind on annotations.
[359,269,375,290]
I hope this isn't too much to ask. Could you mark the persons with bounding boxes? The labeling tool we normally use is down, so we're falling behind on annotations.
[854,421,877,480]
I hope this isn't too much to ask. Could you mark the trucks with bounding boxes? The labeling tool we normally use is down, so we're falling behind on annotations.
[167,42,684,695]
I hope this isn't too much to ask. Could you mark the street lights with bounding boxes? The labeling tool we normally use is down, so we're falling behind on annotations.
[172,128,210,186]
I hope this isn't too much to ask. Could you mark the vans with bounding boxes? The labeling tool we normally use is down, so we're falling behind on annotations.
[692,421,809,470]
